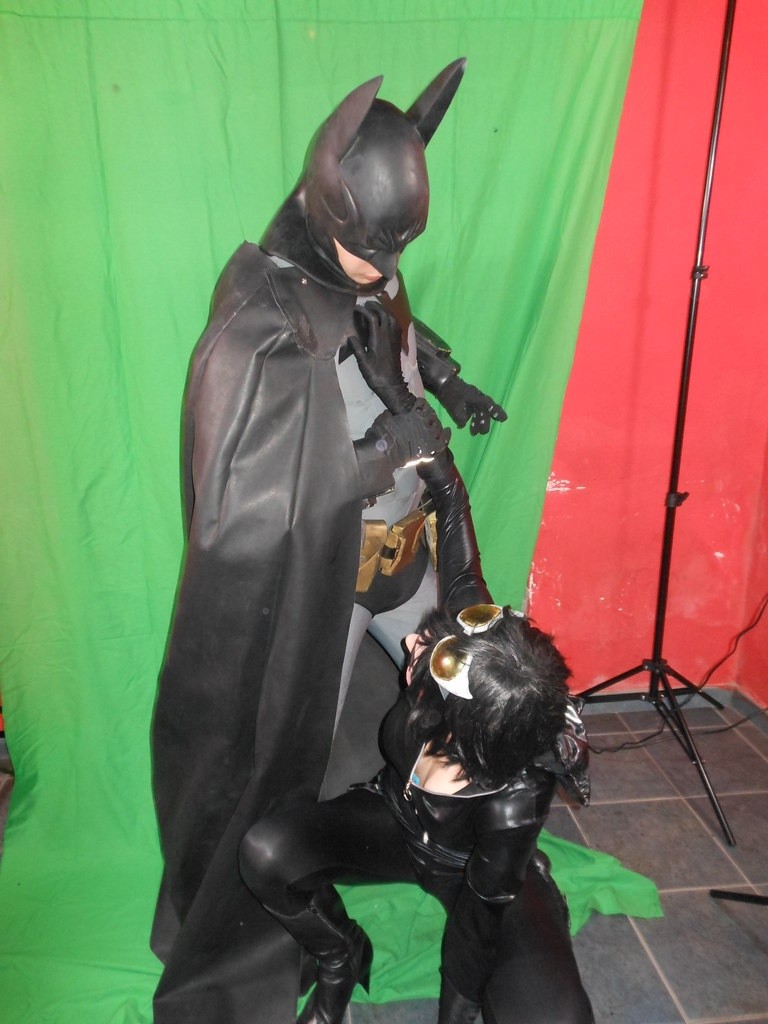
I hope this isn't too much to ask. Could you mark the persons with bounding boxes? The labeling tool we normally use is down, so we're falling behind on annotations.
[244,299,593,1024]
[149,52,507,1024]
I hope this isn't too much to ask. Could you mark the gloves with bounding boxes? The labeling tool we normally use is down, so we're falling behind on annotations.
[370,397,452,469]
[437,377,508,436]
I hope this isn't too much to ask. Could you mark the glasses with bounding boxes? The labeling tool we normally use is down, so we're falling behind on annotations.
[428,603,527,699]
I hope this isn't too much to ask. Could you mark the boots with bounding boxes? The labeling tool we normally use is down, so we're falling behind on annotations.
[263,875,372,1024]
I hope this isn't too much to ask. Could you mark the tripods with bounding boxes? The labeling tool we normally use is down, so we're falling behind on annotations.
[566,0,738,848]
